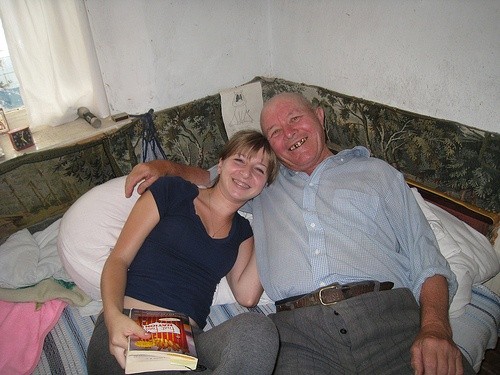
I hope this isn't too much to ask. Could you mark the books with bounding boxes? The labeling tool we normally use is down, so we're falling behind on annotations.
[124,308,199,374]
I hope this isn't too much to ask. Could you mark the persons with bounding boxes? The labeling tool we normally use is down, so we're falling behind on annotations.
[125,91,475,375]
[88,130,280,375]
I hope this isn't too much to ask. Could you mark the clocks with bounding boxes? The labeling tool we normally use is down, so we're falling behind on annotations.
[9,126,34,151]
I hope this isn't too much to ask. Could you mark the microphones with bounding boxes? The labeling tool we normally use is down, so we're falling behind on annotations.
[77,107,101,128]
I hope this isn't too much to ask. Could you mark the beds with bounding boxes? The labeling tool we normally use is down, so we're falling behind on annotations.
[0,179,500,375]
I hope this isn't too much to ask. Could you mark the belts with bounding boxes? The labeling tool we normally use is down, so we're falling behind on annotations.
[275,280,394,312]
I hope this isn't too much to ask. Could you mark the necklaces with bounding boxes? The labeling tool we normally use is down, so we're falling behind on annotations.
[208,191,227,237]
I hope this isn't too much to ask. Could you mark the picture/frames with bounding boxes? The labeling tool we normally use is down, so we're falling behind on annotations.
[0,108,10,135]
